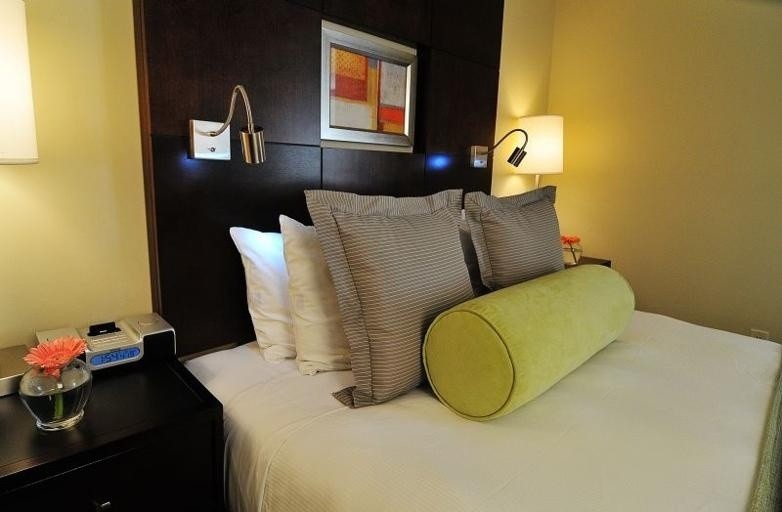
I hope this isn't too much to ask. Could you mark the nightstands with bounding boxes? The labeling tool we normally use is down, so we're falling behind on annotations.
[0,357,224,512]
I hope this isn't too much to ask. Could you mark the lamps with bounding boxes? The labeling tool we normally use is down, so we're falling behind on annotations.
[469,114,564,188]
[187,82,266,166]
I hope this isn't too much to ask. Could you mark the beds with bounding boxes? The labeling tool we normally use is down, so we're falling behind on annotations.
[183,311,781,512]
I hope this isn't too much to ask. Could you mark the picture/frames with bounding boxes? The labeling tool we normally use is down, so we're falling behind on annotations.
[320,24,417,151]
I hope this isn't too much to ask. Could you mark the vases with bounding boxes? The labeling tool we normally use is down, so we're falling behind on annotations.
[16,359,93,431]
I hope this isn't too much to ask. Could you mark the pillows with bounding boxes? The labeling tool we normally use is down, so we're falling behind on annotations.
[275,213,354,377]
[303,185,478,409]
[464,186,564,289]
[230,225,298,364]
[422,262,637,420]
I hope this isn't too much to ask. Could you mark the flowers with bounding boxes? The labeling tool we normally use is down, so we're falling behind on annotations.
[18,331,87,421]
